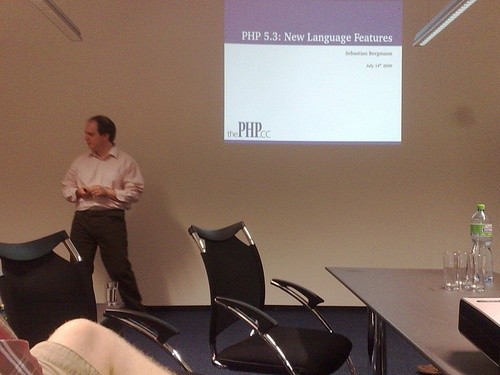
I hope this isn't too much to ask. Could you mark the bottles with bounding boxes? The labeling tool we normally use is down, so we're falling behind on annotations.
[469,204,494,288]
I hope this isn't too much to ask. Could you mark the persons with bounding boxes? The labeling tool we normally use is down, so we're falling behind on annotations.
[62,115,145,315]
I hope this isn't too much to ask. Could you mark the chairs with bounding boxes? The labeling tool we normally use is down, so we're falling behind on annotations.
[0,230,193,374]
[188,222,356,374]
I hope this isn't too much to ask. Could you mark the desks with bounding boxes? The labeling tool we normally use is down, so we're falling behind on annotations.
[325,267,500,375]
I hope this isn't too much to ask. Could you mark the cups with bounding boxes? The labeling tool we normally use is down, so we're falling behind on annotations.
[442,249,486,292]
